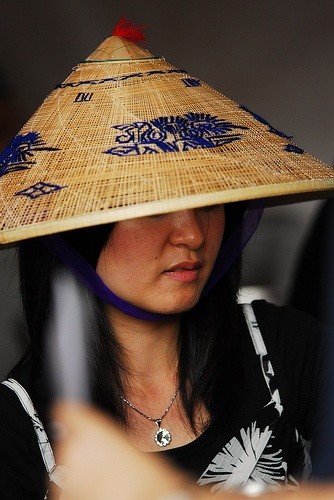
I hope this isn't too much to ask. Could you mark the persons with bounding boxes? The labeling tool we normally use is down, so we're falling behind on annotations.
[0,136,333,500]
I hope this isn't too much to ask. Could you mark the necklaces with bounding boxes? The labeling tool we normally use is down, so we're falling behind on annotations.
[117,384,181,448]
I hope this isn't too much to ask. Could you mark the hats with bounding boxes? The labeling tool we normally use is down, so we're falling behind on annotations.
[1,17,334,249]
